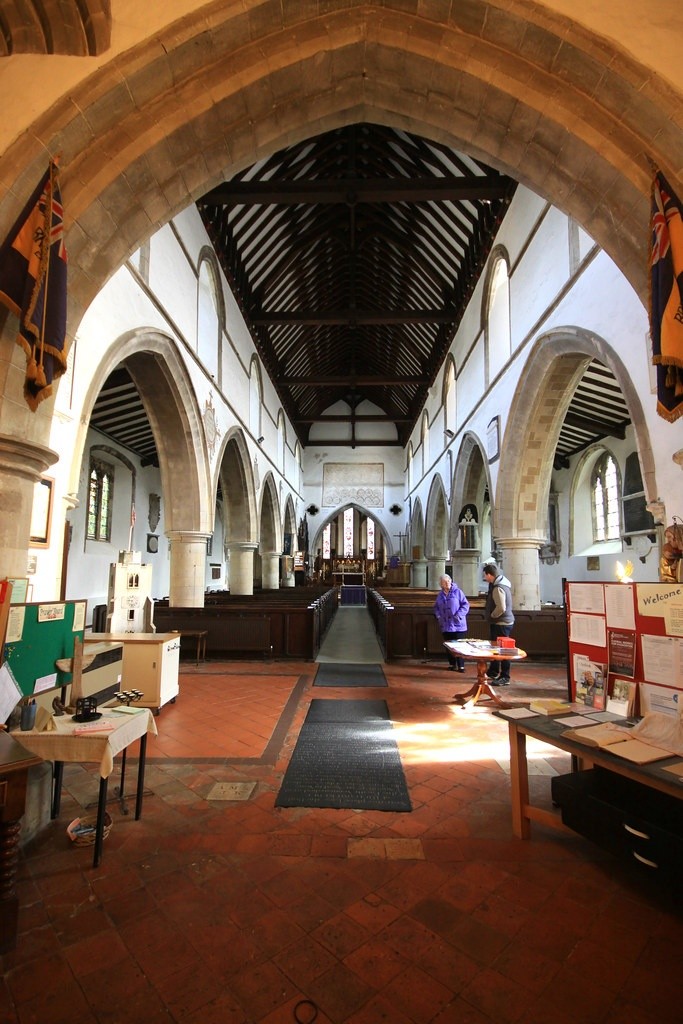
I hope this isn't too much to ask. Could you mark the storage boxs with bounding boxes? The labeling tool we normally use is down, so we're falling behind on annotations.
[552,768,683,901]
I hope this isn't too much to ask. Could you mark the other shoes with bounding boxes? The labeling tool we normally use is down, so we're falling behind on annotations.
[448,665,457,671]
[458,667,465,673]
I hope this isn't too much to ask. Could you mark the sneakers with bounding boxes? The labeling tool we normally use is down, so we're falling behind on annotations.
[491,675,510,685]
[485,673,499,680]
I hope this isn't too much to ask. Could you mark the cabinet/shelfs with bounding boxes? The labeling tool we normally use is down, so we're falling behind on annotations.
[84,633,179,707]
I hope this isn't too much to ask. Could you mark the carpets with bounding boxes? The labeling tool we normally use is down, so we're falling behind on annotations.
[273,698,414,812]
[313,663,389,687]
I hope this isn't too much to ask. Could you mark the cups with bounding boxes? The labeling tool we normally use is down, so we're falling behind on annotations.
[20,704,38,731]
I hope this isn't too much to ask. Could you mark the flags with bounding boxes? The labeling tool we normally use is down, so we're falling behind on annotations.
[649,171,682,423]
[0,157,68,412]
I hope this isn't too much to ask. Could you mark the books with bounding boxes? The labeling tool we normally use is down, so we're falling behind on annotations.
[530,700,571,716]
[561,722,675,766]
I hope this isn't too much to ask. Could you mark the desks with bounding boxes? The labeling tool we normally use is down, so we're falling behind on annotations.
[491,705,683,841]
[169,629,208,667]
[9,705,158,868]
[443,639,528,710]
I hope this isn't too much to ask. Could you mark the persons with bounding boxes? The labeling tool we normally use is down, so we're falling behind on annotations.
[581,671,596,692]
[483,565,515,686]
[661,523,683,582]
[434,574,470,672]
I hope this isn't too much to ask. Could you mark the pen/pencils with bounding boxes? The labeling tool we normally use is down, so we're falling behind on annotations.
[24,696,35,706]
